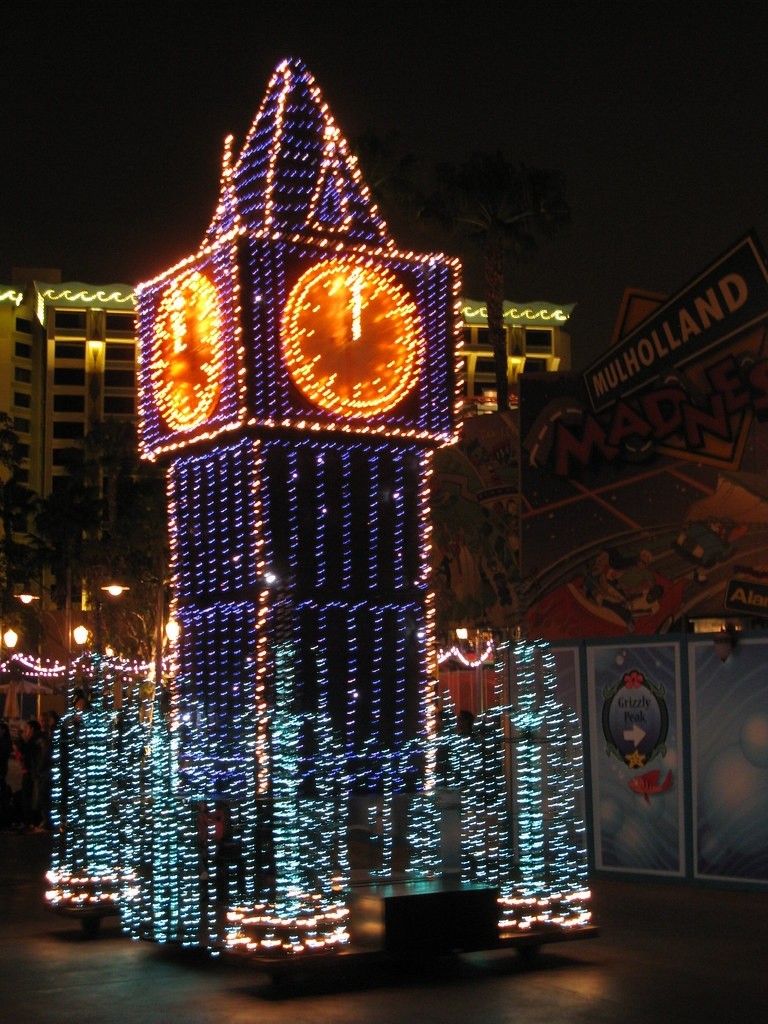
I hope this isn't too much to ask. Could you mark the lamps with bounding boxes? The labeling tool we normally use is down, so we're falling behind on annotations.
[712,628,735,662]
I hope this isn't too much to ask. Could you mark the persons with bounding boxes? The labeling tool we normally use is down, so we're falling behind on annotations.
[0,690,96,834]
[436,709,474,778]
